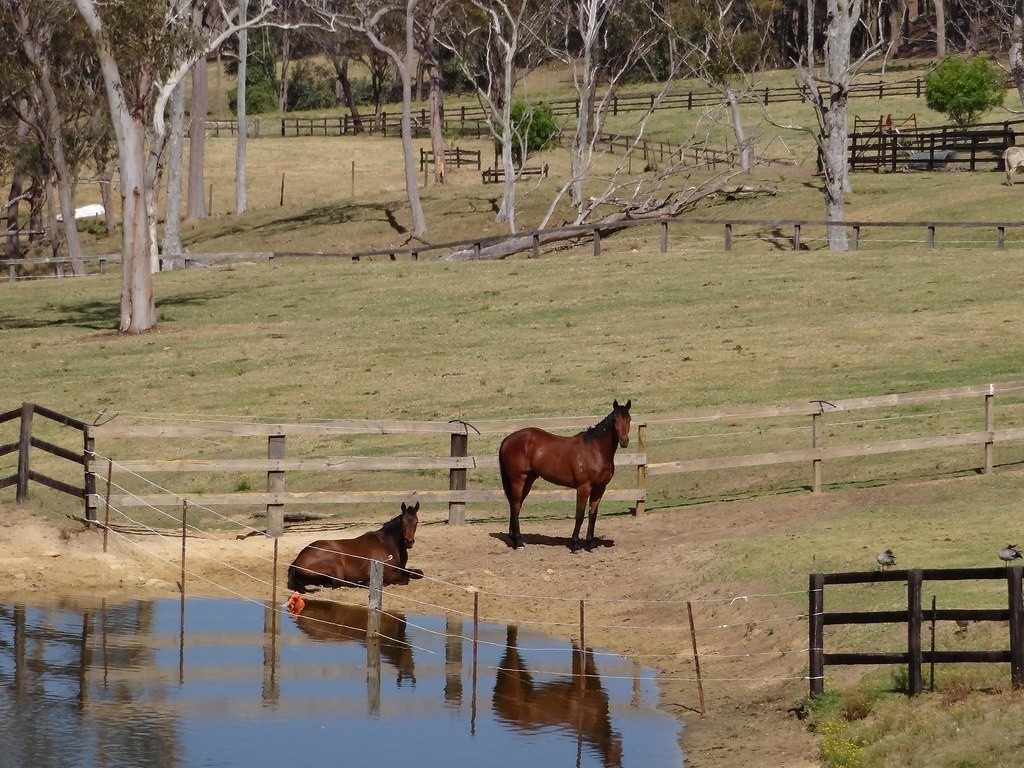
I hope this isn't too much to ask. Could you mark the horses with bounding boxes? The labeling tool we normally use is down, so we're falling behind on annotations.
[499,399,632,555]
[287,501,424,594]
[1002,147,1024,185]
